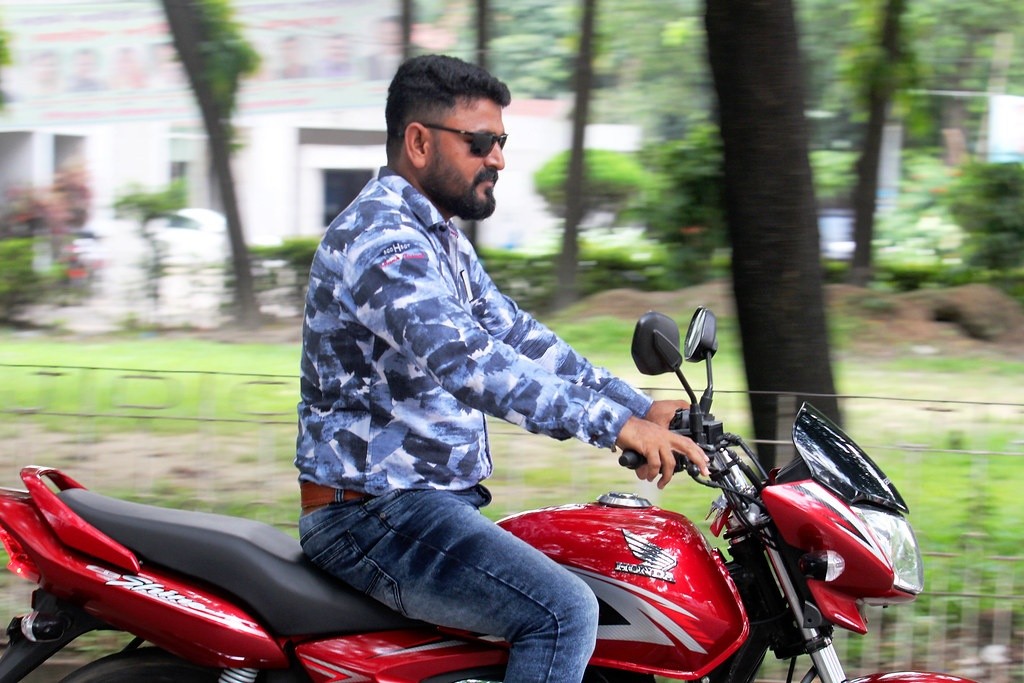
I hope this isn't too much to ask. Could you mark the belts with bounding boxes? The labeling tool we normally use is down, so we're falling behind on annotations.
[301,481,365,509]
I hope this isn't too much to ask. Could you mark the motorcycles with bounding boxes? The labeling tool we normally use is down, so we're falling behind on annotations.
[1,307,978,682]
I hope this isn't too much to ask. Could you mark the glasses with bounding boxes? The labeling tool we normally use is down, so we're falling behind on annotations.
[399,124,508,157]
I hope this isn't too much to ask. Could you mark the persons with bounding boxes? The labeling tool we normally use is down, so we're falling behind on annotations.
[294,54,710,683]
